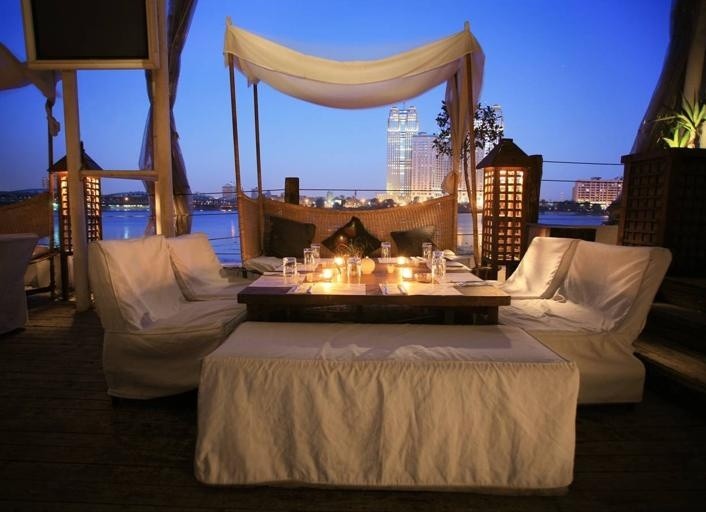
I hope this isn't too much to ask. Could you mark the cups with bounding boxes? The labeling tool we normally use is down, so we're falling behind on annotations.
[347,257,362,277]
[311,243,320,258]
[381,242,391,263]
[283,257,297,277]
[303,248,314,265]
[422,243,432,260]
[432,259,445,282]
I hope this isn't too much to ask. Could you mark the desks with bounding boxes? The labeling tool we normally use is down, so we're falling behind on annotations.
[237,271,511,325]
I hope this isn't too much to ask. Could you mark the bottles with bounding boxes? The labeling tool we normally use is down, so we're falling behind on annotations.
[414,262,432,283]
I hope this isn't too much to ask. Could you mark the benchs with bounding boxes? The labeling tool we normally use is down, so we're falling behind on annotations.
[222,16,486,278]
[0,41,61,312]
[194,320,579,496]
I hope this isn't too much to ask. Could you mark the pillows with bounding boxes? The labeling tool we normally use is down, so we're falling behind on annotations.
[322,216,385,260]
[262,215,315,261]
[389,223,445,257]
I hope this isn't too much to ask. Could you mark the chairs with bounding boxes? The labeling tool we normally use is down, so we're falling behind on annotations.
[166,232,255,302]
[1,232,41,336]
[86,235,246,401]
[498,240,672,405]
[496,236,577,299]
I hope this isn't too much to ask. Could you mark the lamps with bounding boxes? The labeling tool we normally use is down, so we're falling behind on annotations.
[46,141,104,255]
[470,138,530,280]
